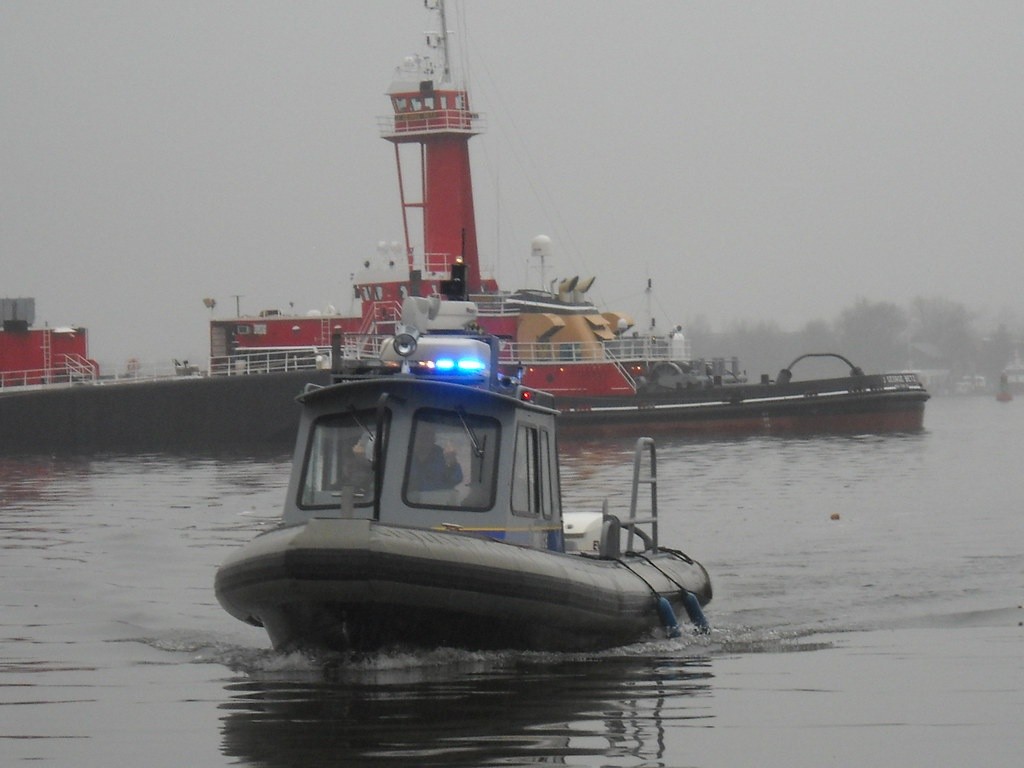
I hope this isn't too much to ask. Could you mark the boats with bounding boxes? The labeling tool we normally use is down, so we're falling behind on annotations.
[215,330,716,654]
[558,355,934,436]
[1,0,747,446]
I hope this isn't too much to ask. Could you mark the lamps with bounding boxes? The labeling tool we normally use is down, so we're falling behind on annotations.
[393,335,417,373]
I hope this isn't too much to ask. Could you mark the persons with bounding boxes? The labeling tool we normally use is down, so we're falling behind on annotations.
[321,455,374,493]
[672,326,684,357]
[405,426,463,494]
